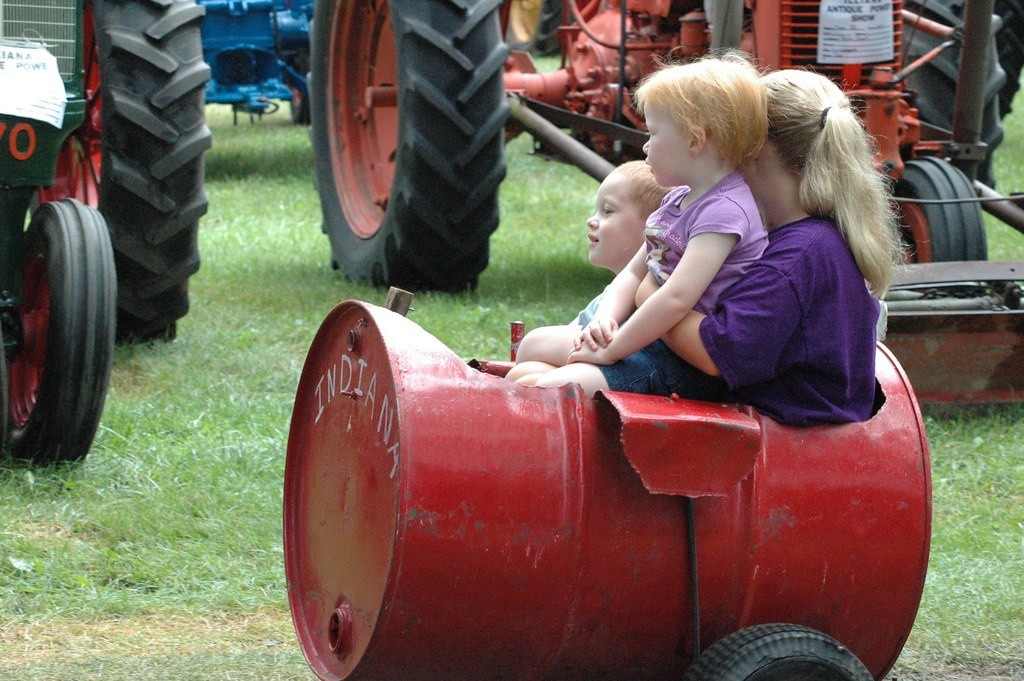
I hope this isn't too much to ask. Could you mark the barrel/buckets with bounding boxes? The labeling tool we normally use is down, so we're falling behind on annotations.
[284,299,933,681]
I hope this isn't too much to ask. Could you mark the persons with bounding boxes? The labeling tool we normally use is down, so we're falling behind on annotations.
[571,161,674,324]
[504,69,895,425]
[514,57,770,396]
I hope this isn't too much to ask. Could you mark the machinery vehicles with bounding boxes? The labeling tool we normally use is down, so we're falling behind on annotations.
[0,2,1024,472]
